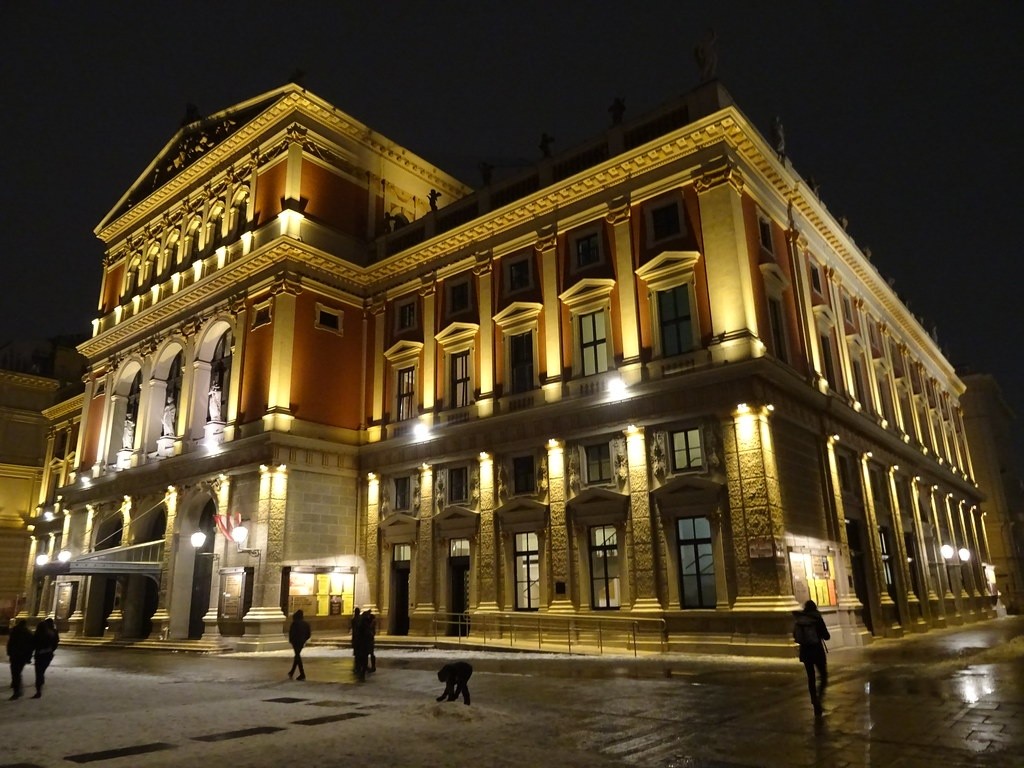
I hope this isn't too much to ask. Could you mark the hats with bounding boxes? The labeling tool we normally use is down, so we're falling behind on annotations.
[804,600,817,611]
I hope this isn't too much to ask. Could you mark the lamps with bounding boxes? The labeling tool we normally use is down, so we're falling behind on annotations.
[231,522,262,557]
[190,528,220,562]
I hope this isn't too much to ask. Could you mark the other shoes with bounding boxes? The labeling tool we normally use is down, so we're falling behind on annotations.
[368,667,376,672]
[296,673,306,680]
[813,702,824,717]
[462,700,470,705]
[436,695,447,701]
[287,670,294,677]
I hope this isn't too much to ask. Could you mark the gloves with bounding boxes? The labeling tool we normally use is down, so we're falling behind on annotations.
[448,694,459,701]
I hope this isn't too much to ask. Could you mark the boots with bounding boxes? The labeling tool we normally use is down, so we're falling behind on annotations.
[31,680,42,698]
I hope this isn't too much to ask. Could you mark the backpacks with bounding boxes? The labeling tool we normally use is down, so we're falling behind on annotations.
[791,611,828,653]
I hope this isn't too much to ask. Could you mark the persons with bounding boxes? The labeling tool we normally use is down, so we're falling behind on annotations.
[208,382,221,422]
[793,601,830,716]
[351,607,376,680]
[6,618,61,700]
[288,610,311,680]
[123,414,135,449]
[160,397,176,435]
[436,662,473,705]
